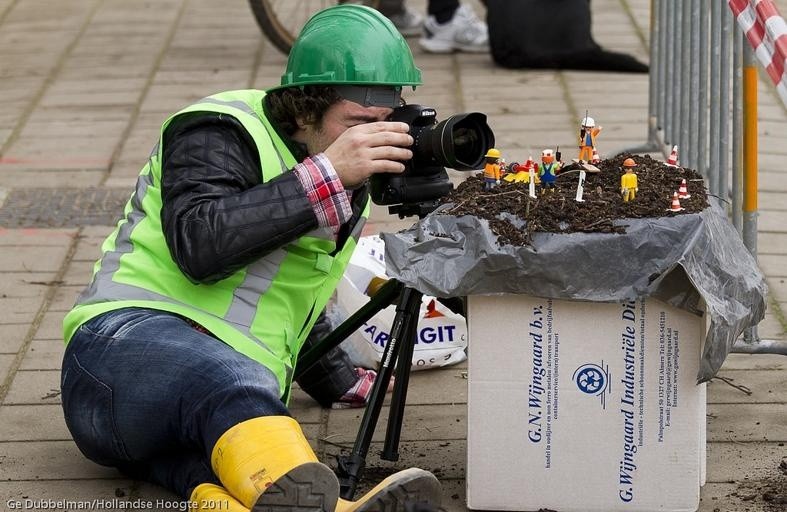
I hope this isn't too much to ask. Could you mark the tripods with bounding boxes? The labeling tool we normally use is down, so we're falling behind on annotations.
[294,206,442,501]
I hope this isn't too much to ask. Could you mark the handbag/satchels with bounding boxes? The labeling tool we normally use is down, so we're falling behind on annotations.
[334,235,467,372]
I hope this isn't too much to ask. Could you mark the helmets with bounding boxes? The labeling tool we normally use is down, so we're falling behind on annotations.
[265,4,424,92]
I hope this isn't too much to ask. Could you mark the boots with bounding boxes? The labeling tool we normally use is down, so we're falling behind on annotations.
[211,415,442,512]
[189,462,339,512]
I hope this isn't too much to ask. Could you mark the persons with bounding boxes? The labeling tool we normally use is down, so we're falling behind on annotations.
[477,1,649,75]
[56,1,445,511]
[372,1,493,56]
[481,110,692,214]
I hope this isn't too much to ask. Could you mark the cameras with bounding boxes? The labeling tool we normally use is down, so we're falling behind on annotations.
[369,103,496,206]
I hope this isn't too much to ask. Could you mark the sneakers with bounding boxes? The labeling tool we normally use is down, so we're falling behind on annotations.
[387,8,424,35]
[419,4,489,52]
